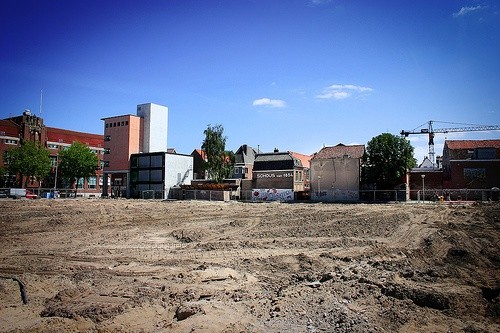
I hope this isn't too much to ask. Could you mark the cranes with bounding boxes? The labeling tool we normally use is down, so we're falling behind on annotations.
[400,119,500,166]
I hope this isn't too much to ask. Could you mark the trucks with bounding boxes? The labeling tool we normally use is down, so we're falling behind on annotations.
[9,188,38,200]
